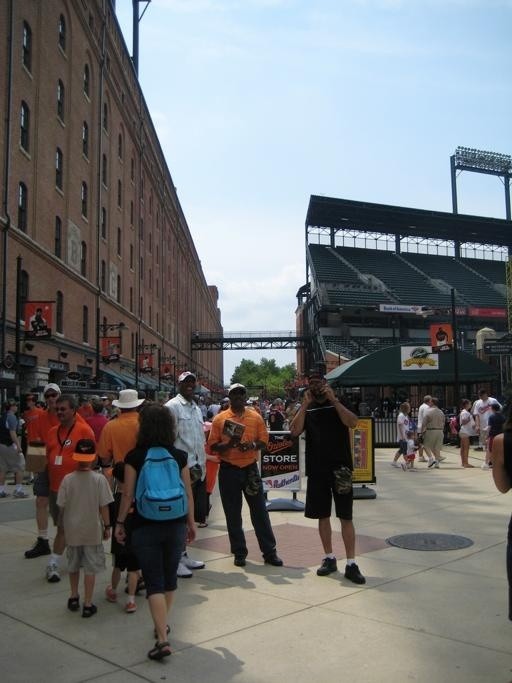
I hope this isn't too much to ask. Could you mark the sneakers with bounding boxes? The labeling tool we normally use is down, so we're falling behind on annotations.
[177,550,205,577]
[345,563,365,584]
[46,561,145,617]
[235,554,246,566]
[0,485,29,498]
[263,551,282,565]
[25,537,50,557]
[392,456,446,471]
[317,557,336,575]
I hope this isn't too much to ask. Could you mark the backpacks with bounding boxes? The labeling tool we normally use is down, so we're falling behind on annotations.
[135,447,188,523]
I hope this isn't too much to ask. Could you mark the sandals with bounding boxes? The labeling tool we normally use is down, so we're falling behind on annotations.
[148,624,170,658]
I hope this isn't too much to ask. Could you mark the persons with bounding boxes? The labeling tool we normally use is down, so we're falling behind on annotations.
[491,406,511,621]
[29,308,47,331]
[104,462,142,612]
[72,390,119,440]
[116,401,196,657]
[25,382,61,559]
[458,399,478,467]
[0,394,46,498]
[436,327,449,346]
[43,394,95,582]
[473,389,502,467]
[421,397,445,468]
[208,382,284,567]
[55,438,114,617]
[392,402,411,468]
[200,421,219,503]
[287,373,367,584]
[416,395,445,462]
[266,388,301,430]
[97,388,147,590]
[196,395,230,422]
[402,430,419,471]
[163,371,208,578]
[485,403,504,468]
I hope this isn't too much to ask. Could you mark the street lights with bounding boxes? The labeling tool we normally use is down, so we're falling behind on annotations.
[421,303,461,441]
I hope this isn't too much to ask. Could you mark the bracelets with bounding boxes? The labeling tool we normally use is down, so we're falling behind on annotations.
[116,520,124,525]
[104,525,111,528]
[253,442,257,449]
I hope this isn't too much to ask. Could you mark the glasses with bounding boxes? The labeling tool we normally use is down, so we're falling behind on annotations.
[44,394,57,398]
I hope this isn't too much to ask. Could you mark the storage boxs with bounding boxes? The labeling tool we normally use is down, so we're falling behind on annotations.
[26,442,48,473]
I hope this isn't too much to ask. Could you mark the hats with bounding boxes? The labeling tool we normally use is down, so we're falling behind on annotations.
[112,389,145,408]
[178,371,197,384]
[228,383,245,395]
[73,439,96,462]
[44,383,61,393]
